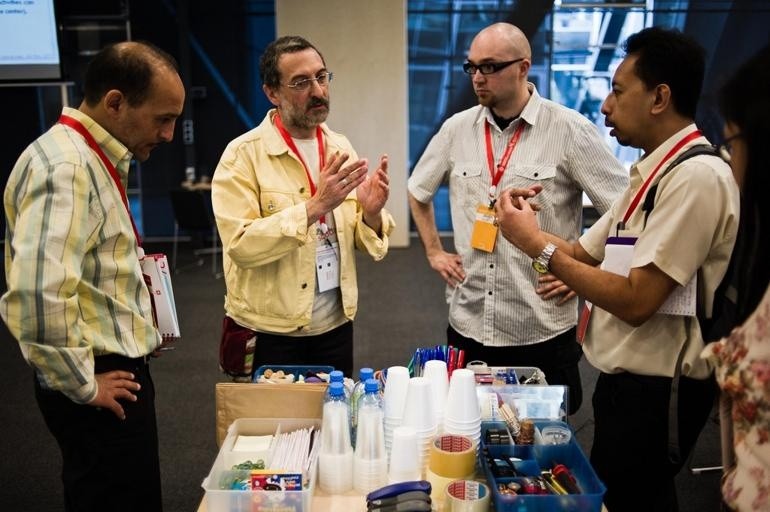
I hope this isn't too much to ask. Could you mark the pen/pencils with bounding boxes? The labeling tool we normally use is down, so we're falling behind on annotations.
[413,344,465,383]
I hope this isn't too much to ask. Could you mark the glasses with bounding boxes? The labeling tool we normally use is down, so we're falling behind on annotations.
[279,72,335,89]
[461,57,524,75]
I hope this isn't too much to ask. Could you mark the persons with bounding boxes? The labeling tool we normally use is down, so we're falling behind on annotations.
[495,26,741,512]
[406,21,629,418]
[0,40,184,512]
[211,36,396,379]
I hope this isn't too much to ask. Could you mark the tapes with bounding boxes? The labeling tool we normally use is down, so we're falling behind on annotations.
[443,478,491,512]
[426,467,476,502]
[429,433,476,478]
[430,494,452,512]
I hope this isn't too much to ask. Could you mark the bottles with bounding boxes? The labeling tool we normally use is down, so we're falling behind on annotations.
[349,367,383,452]
[356,379,384,454]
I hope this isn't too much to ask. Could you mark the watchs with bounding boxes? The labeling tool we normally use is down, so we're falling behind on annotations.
[530,242,558,276]
